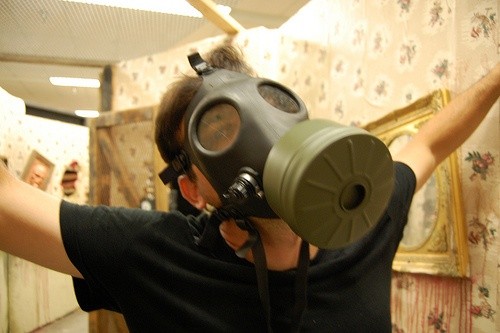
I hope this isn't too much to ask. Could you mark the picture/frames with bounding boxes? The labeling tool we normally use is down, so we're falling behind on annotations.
[360,88,471,279]
[21,149,55,191]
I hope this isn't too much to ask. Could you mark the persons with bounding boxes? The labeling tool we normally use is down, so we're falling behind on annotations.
[0,43,500,333]
